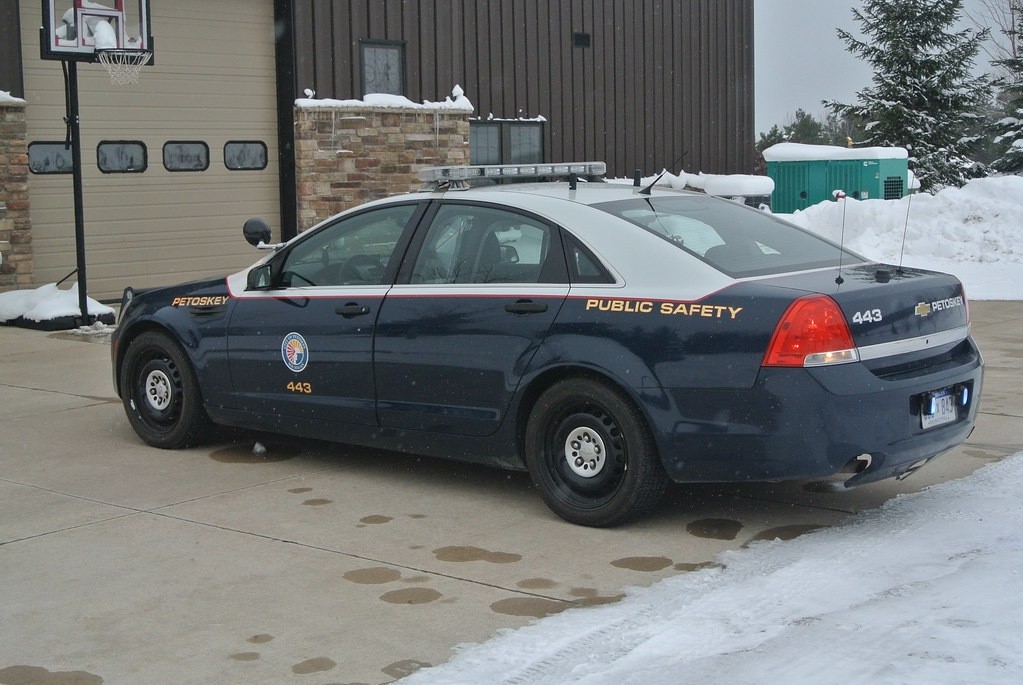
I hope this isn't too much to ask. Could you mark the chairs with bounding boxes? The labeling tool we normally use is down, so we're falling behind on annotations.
[407,231,770,291]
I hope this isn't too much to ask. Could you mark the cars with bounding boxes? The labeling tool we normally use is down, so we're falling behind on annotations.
[112,147,985,527]
